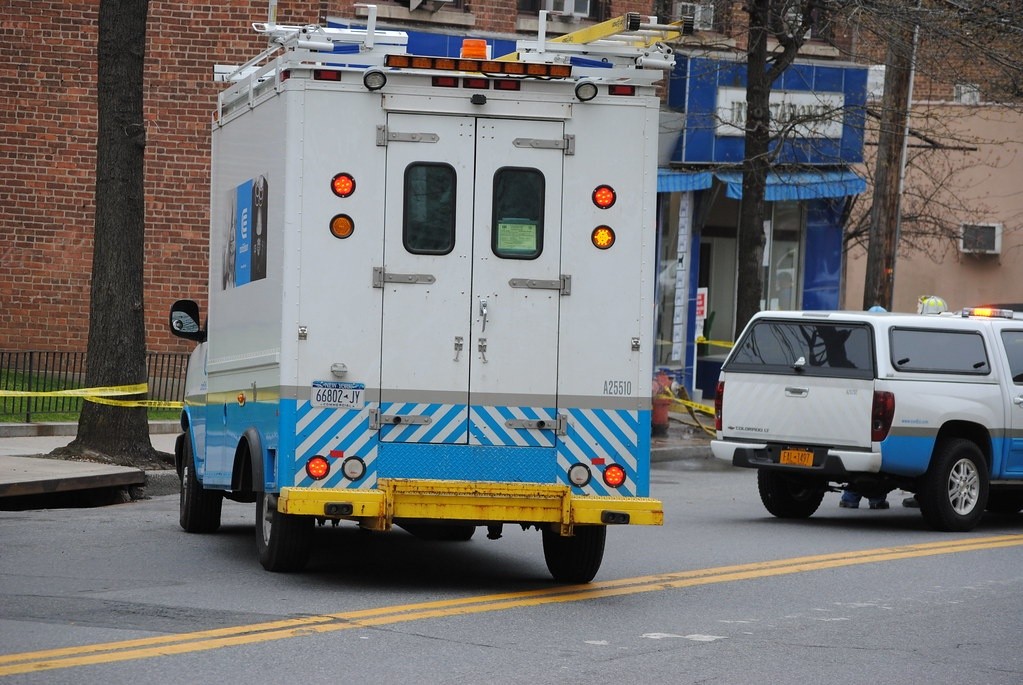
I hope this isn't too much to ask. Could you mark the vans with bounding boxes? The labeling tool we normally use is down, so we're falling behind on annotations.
[709,306,1023,533]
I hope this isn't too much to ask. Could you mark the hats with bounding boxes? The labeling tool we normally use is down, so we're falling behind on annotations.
[869,307,885,313]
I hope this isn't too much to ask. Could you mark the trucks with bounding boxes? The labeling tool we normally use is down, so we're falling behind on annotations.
[169,2,679,584]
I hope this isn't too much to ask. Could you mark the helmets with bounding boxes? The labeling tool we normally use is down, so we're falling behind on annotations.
[916,295,947,314]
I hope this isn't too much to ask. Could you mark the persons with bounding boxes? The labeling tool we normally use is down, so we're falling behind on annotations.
[902,295,948,508]
[839,306,889,510]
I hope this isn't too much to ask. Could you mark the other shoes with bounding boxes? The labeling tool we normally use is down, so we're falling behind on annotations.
[903,497,920,508]
[870,500,890,509]
[838,500,859,508]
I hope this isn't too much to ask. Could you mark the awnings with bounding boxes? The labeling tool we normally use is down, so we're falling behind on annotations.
[715,168,867,201]
[657,167,712,192]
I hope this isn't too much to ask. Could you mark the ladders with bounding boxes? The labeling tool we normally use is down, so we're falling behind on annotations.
[491,12,695,63]
